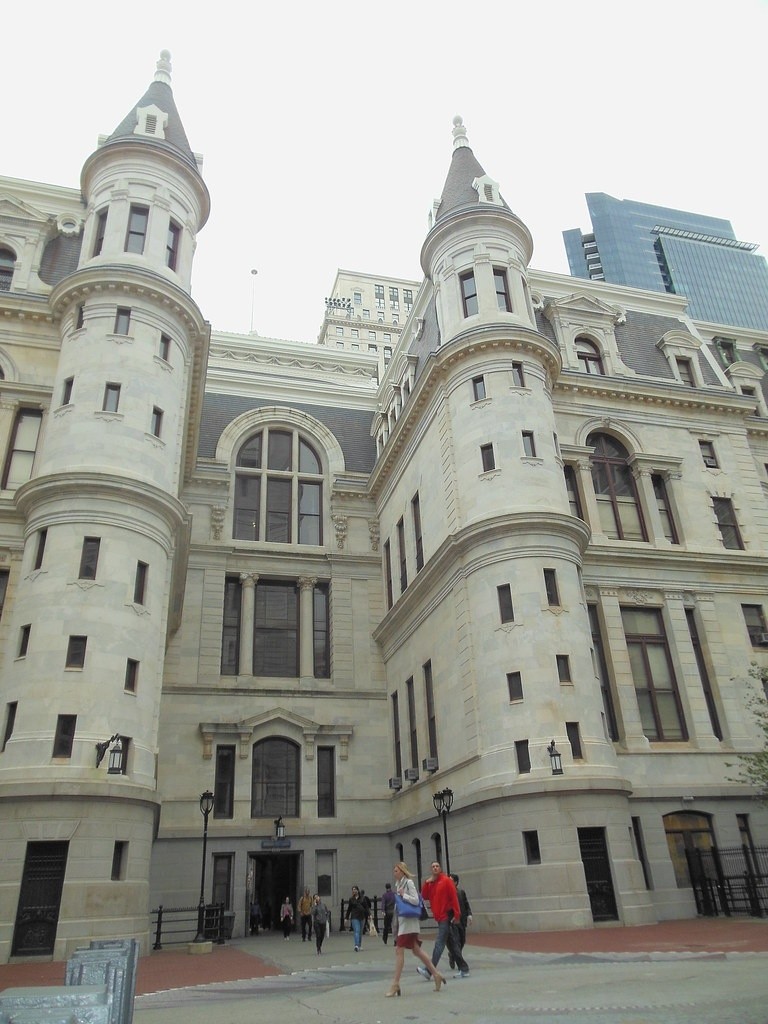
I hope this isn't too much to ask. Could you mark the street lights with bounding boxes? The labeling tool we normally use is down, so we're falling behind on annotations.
[193,790,214,942]
[432,788,454,875]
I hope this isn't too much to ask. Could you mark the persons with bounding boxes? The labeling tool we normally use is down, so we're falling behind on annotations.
[250,898,272,936]
[381,883,396,944]
[385,862,447,997]
[281,896,294,942]
[297,888,314,942]
[310,894,330,955]
[360,889,371,935]
[448,874,473,972]
[417,860,471,982]
[344,885,372,952]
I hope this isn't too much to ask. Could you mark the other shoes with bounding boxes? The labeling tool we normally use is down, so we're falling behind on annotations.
[308,937,311,941]
[284,938,286,941]
[448,952,455,970]
[317,950,321,955]
[418,940,422,947]
[360,947,363,950]
[287,936,289,941]
[354,946,359,952]
[302,939,305,942]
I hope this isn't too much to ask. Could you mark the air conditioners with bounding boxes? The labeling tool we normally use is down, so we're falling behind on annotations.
[704,459,717,467]
[405,769,419,781]
[423,758,437,771]
[389,777,402,788]
[754,633,768,645]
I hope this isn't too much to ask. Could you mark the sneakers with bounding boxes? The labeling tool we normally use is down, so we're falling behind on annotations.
[416,967,431,980]
[452,970,470,977]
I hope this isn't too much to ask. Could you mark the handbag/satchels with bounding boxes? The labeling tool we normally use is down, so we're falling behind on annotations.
[291,919,294,924]
[395,880,422,917]
[369,918,378,938]
[417,901,428,921]
[325,920,329,939]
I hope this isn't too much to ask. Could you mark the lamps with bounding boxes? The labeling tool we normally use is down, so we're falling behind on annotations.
[274,816,285,838]
[95,733,123,774]
[547,739,563,775]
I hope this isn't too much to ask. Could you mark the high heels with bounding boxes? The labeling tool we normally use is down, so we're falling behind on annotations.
[386,985,401,997]
[433,972,447,991]
